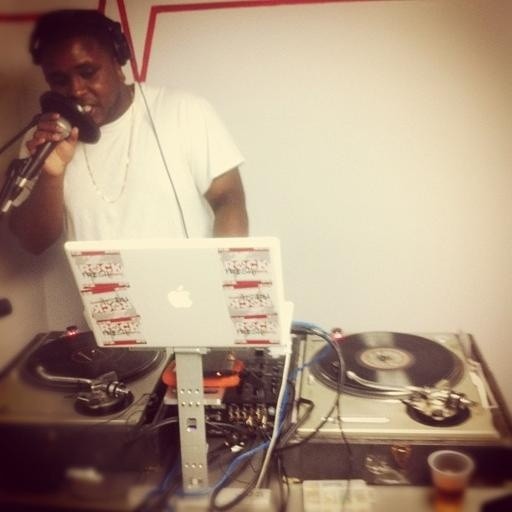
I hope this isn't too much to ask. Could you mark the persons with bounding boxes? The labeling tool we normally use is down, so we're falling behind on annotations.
[1,9,249,255]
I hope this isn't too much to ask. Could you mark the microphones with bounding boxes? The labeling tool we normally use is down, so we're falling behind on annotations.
[0,118,72,214]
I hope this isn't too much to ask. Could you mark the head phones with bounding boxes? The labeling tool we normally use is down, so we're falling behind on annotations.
[29,10,131,66]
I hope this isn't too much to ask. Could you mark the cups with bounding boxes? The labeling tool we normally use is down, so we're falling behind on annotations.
[426,449,475,512]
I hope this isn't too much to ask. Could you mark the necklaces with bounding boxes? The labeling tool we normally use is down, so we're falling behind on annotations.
[81,85,136,206]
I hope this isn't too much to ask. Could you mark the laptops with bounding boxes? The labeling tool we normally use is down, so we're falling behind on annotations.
[63,236,294,348]
